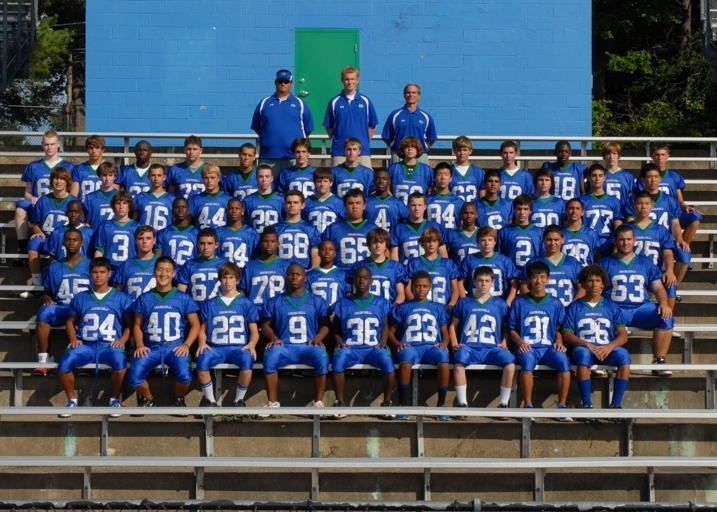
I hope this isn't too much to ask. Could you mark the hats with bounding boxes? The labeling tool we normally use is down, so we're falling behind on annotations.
[274,72,293,85]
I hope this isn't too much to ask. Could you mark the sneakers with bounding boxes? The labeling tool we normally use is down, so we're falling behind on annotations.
[13,249,23,265]
[194,395,217,419]
[495,403,509,420]
[108,397,121,417]
[305,399,333,418]
[173,396,186,407]
[58,399,78,417]
[137,396,159,407]
[555,406,573,422]
[650,357,672,377]
[607,400,632,422]
[32,367,48,376]
[582,404,601,426]
[395,403,410,420]
[257,401,280,417]
[377,399,395,419]
[333,399,347,418]
[19,290,43,300]
[454,401,469,421]
[430,415,451,421]
[231,398,249,418]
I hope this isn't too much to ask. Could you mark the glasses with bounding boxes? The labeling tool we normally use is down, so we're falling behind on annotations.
[276,80,290,84]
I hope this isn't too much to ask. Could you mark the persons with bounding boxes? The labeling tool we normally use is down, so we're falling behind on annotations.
[324,68,378,168]
[13,133,704,422]
[251,69,314,168]
[382,83,437,165]
